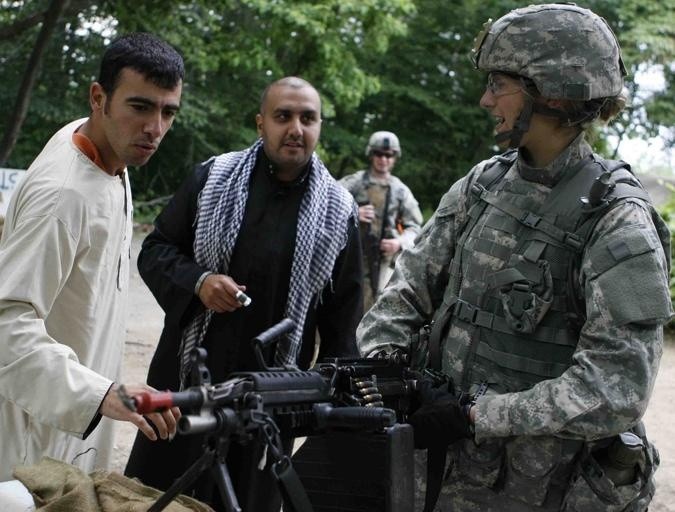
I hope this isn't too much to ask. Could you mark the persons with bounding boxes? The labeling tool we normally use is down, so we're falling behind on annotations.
[124,76,365,512]
[354,3,675,512]
[337,130,426,302]
[0,31,183,479]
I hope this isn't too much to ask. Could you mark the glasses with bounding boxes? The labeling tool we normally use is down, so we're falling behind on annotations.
[487,72,526,98]
[371,149,395,158]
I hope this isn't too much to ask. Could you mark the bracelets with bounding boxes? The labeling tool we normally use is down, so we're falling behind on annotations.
[194,271,212,296]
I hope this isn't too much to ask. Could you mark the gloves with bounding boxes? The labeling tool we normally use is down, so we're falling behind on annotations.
[404,386,475,450]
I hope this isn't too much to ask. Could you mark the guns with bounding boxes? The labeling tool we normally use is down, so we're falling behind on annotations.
[118,316,453,511]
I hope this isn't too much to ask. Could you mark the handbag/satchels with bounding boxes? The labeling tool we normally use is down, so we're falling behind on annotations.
[560,435,662,510]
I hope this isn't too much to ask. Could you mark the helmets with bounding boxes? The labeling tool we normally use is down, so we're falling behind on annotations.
[470,1,623,104]
[365,131,403,157]
